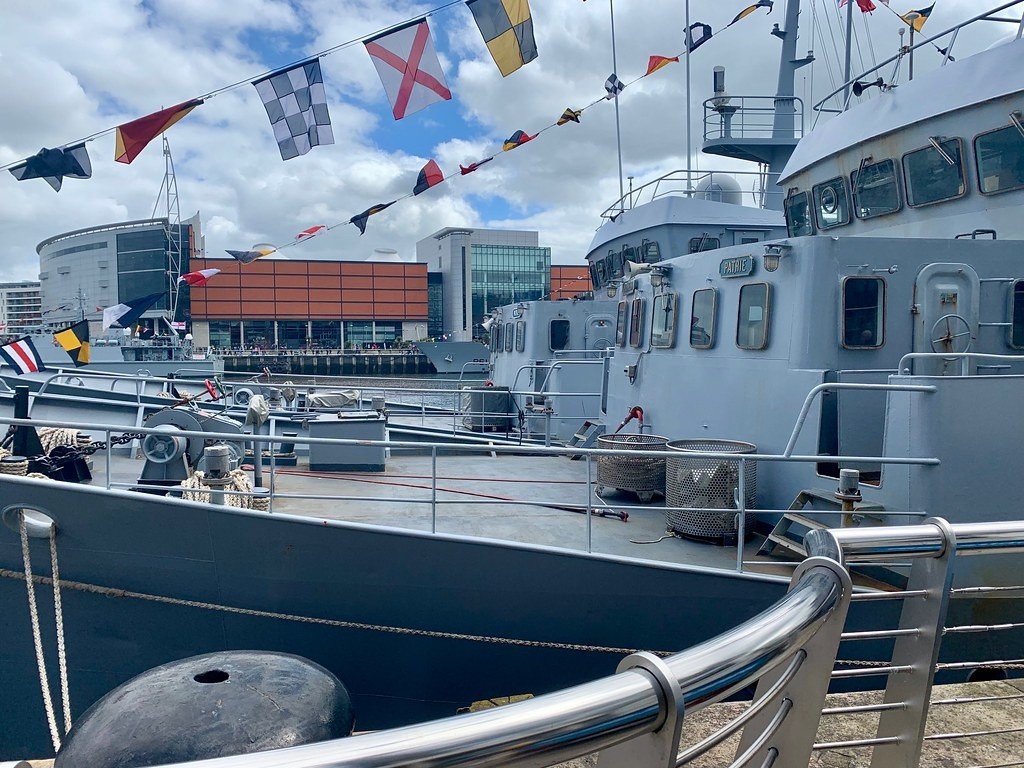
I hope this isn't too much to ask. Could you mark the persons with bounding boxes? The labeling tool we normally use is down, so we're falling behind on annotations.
[211,343,418,355]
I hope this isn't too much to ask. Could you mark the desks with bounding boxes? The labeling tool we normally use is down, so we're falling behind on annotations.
[711,104,741,138]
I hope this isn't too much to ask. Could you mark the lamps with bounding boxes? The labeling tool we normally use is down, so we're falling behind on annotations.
[763,244,791,272]
[650,265,673,287]
[517,303,528,315]
[606,280,623,298]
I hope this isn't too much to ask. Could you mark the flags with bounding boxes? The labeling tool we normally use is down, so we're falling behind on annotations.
[7,142,91,192]
[728,0,774,27]
[0,336,46,376]
[459,157,493,175]
[103,291,165,332]
[362,17,452,120]
[413,159,444,196]
[645,56,679,75]
[604,73,625,100]
[349,200,397,236]
[114,98,198,165]
[225,243,278,265]
[838,0,936,32]
[466,0,538,77]
[253,57,335,161]
[557,108,582,126]
[295,224,328,242]
[177,269,221,286]
[431,334,447,343]
[503,130,538,151]
[52,319,89,368]
[683,22,713,52]
[171,322,185,330]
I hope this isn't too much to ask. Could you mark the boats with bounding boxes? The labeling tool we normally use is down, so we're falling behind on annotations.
[2,1,1024,768]
[0,287,226,379]
[418,342,491,374]
